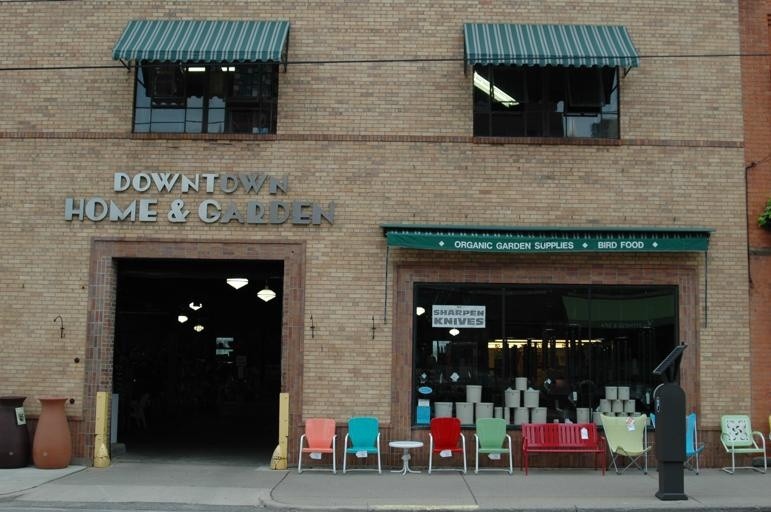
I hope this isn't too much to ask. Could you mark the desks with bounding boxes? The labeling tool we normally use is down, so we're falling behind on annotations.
[388,440,424,476]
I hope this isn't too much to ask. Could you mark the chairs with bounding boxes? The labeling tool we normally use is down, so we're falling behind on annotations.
[428,417,467,474]
[650,413,705,475]
[719,415,767,474]
[600,414,652,475]
[298,417,338,475]
[473,418,513,475]
[342,416,382,474]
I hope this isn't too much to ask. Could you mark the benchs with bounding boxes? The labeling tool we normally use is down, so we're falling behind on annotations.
[520,423,606,476]
